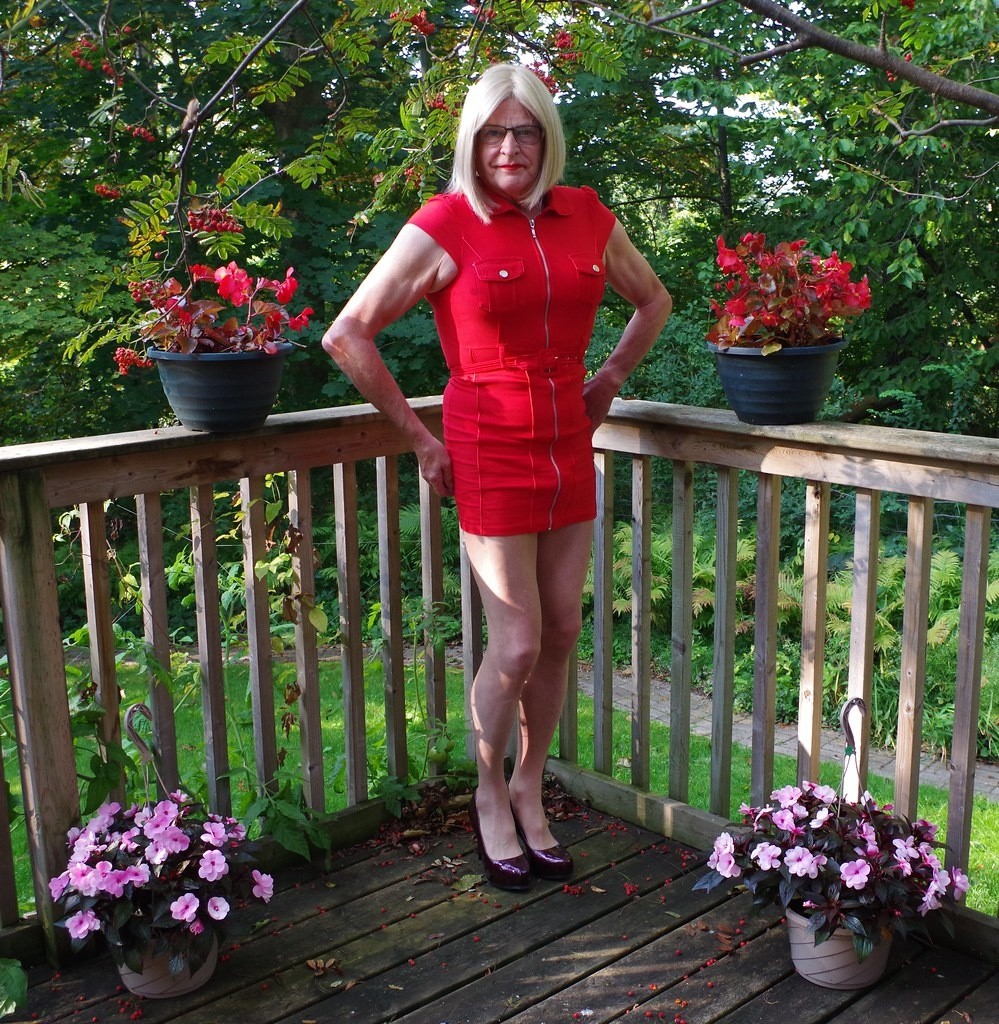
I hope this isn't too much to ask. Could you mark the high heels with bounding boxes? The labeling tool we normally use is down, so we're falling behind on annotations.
[506,778,573,881]
[467,785,534,890]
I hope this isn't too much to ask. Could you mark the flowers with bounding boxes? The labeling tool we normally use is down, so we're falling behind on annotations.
[706,232,872,357]
[142,262,314,354]
[690,780,970,964]
[49,788,273,977]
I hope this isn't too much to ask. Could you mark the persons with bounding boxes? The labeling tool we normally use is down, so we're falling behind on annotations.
[321,64,673,892]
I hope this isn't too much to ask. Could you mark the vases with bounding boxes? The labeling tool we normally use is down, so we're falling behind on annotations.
[786,899,894,992]
[116,933,219,999]
[710,341,851,424]
[147,343,297,434]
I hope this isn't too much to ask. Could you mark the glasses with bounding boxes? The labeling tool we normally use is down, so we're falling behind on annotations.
[477,123,545,146]
